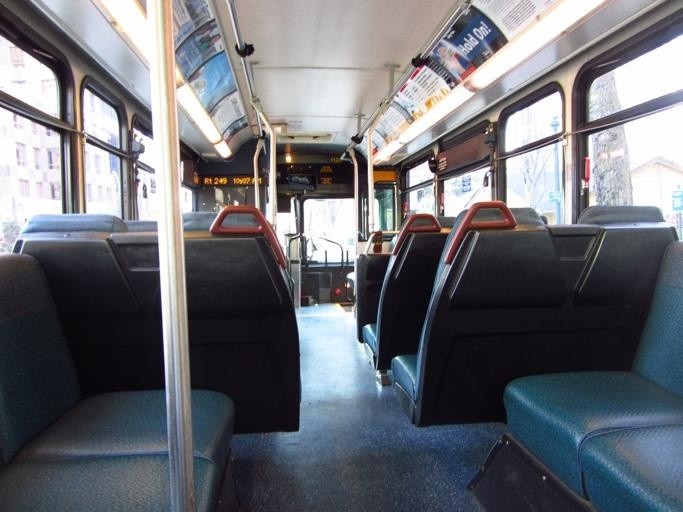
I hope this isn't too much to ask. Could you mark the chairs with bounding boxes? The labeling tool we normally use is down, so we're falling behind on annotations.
[554,200,678,374]
[10,202,302,434]
[389,202,554,426]
[353,200,550,383]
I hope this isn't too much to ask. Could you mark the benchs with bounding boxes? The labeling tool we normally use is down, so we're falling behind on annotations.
[0,253,242,511]
[465,241,683,512]
